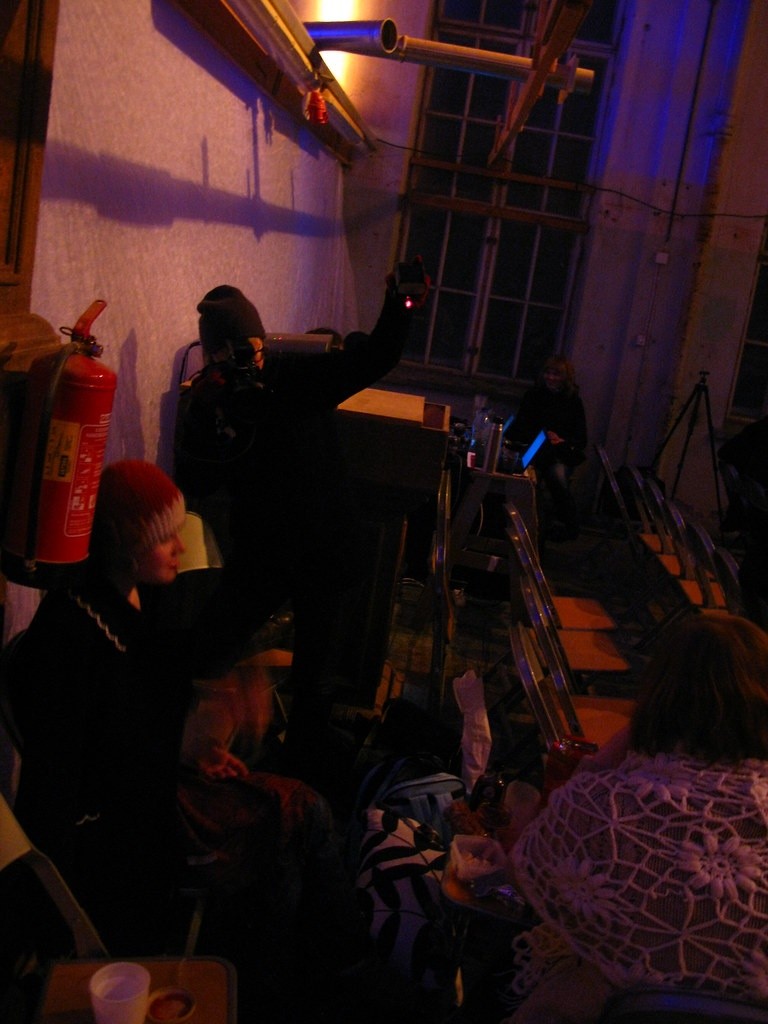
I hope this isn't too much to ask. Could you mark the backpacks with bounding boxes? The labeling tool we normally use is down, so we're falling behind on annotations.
[350,748,468,845]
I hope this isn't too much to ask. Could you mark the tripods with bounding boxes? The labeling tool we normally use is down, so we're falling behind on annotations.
[648,371,725,550]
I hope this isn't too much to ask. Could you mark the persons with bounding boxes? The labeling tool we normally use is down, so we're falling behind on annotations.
[174,253,432,783]
[0,459,379,965]
[507,353,587,519]
[495,610,768,1024]
[718,413,768,634]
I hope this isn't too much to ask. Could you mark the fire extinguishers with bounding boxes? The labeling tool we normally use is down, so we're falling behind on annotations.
[0,300,119,587]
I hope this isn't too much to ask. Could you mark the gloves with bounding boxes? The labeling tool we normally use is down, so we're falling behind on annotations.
[381,254,431,322]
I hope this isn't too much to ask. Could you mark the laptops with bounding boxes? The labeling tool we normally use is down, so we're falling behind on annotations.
[496,428,549,475]
[502,414,515,434]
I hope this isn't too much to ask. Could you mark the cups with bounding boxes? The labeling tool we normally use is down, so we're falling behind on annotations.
[146,985,200,1024]
[89,961,149,1024]
[477,801,522,852]
[504,780,542,833]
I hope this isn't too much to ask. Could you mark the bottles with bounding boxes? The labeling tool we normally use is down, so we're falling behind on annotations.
[480,416,505,473]
[467,757,505,813]
[466,407,490,469]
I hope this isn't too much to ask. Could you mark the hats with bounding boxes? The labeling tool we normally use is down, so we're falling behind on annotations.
[94,459,186,559]
[197,285,270,367]
[544,357,568,377]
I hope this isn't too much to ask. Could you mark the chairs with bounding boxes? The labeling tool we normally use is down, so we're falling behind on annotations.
[609,464,676,593]
[491,527,632,712]
[686,521,723,635]
[622,498,728,657]
[491,577,639,749]
[592,439,644,562]
[0,791,238,1024]
[482,501,620,712]
[712,547,747,620]
[613,477,717,626]
[503,622,566,780]
